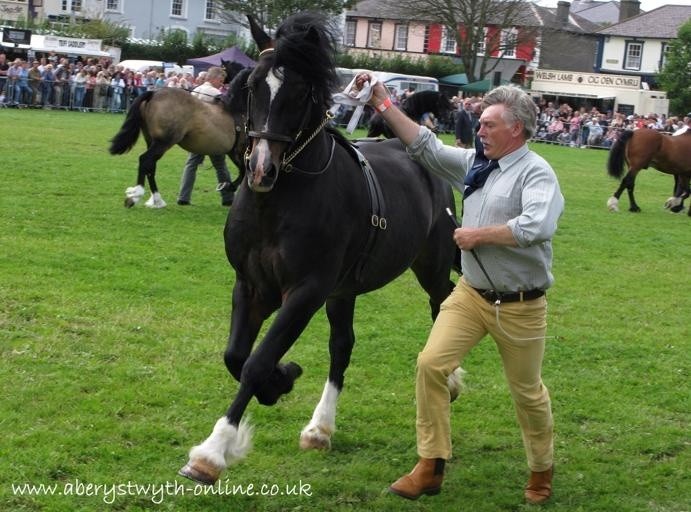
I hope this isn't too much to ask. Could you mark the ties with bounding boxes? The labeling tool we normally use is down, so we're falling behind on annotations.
[461,134,499,218]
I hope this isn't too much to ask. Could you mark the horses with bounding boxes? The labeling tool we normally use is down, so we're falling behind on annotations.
[177,12,468,487]
[110,65,255,209]
[220,58,245,84]
[607,128,691,214]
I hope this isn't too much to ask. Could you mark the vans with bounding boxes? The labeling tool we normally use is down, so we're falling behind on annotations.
[333,64,441,98]
[115,60,182,77]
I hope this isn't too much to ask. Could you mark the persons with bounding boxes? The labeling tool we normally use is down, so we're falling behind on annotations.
[528,99,691,208]
[334,88,484,148]
[177,66,235,206]
[348,75,568,504]
[0,48,231,113]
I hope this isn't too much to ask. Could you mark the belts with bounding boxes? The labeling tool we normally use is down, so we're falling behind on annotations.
[470,285,545,304]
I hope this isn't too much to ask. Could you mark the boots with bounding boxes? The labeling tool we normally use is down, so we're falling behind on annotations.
[524,465,554,505]
[392,458,445,499]
